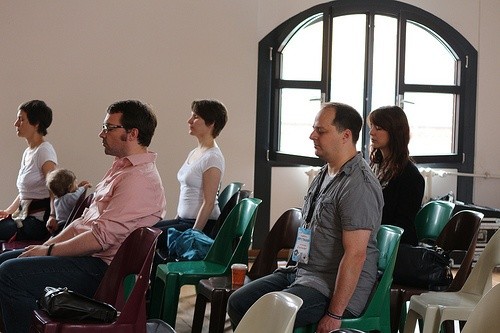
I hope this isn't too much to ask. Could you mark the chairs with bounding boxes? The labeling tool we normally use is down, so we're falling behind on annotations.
[150,198,263,331]
[390,210,484,333]
[32,226,163,333]
[217,182,245,212]
[2,190,87,252]
[404,227,500,333]
[294,225,404,333]
[233,291,303,333]
[461,283,500,333]
[191,207,302,333]
[208,190,253,242]
[415,199,456,246]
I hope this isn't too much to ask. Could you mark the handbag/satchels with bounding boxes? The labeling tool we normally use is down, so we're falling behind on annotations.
[36,286,121,323]
[377,239,455,291]
[167,227,215,262]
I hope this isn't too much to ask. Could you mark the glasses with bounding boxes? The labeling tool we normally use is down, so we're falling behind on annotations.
[103,123,125,134]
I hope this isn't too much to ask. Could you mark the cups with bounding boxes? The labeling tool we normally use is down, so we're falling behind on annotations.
[232,264,248,286]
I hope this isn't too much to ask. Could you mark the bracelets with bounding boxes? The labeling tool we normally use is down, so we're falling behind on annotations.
[324,309,343,321]
[48,213,56,217]
[47,243,54,256]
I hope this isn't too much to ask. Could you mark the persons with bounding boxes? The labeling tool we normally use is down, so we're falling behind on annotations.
[152,98,229,239]
[226,101,385,332]
[365,105,426,239]
[45,167,93,238]
[0,99,59,242]
[1,99,167,332]
